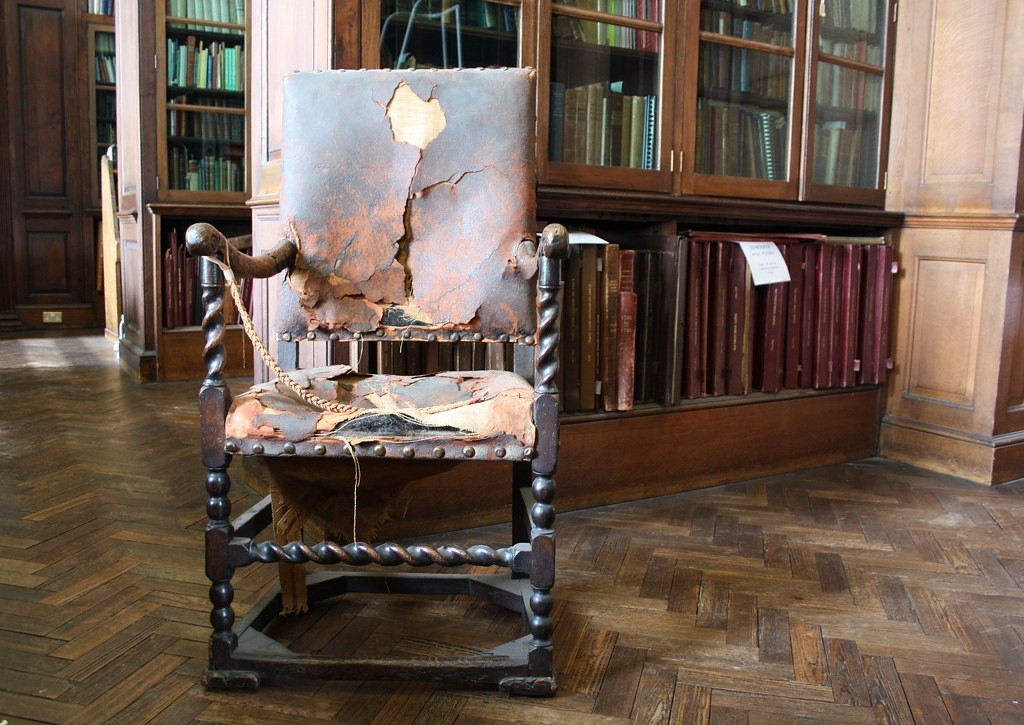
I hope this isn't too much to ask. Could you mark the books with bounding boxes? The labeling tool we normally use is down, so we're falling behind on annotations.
[551,82,565,161]
[85,0,893,410]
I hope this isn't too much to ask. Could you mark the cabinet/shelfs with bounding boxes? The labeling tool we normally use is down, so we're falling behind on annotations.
[81,1,115,210]
[107,1,255,385]
[240,0,904,547]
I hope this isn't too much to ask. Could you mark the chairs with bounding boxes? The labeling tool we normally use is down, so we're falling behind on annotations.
[187,66,572,700]
